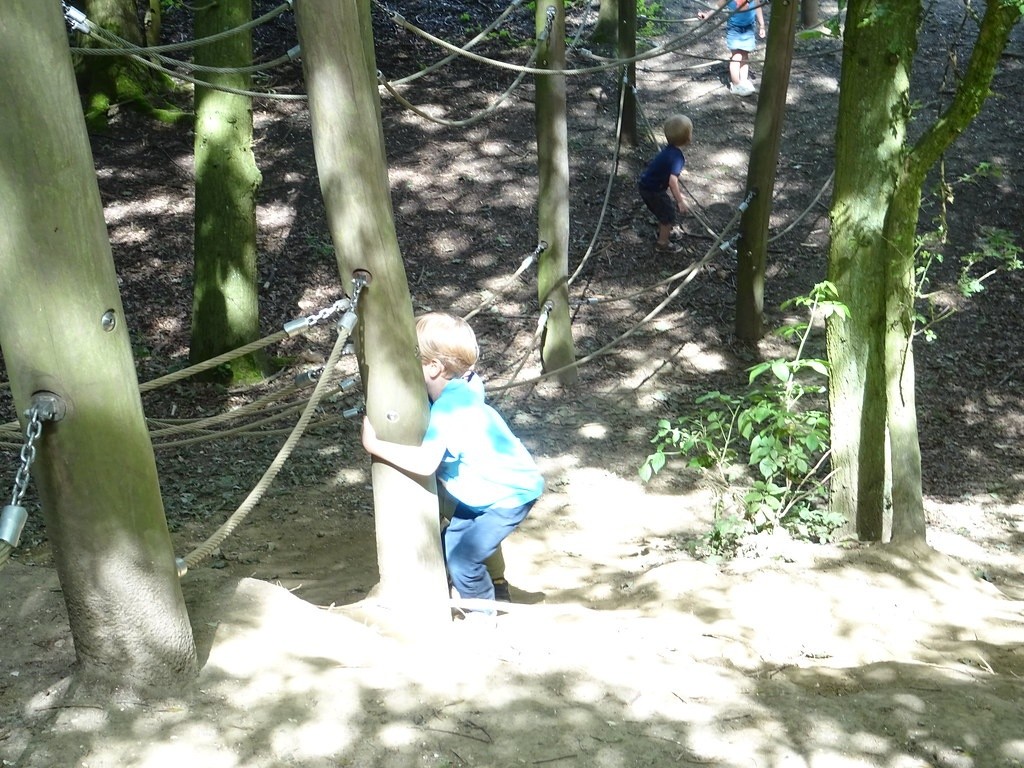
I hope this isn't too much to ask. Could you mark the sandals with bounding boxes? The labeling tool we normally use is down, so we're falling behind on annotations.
[656,241,683,253]
[655,231,683,241]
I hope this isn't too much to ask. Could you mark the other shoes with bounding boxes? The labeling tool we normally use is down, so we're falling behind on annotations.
[729,82,752,96]
[741,80,755,92]
[493,577,510,599]
[456,609,496,636]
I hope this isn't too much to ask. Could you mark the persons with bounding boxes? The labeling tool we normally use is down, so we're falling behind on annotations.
[361,311,544,622]
[697,0,766,97]
[635,115,693,253]
